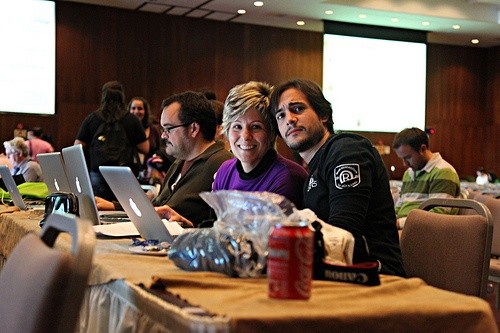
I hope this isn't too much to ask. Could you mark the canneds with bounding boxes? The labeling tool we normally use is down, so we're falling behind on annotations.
[267,221,314,301]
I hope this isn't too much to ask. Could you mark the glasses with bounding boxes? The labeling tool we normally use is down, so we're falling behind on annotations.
[160,121,197,134]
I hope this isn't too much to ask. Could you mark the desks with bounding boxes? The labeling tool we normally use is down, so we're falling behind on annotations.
[0,203,499,333]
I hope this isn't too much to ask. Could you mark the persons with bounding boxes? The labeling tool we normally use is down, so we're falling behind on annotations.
[476,168,488,185]
[4,136,42,182]
[74,81,149,202]
[25,126,53,163]
[14,122,26,139]
[211,101,231,152]
[128,97,159,176]
[95,90,233,228]
[488,170,500,183]
[393,127,461,229]
[211,79,407,278]
[154,81,309,228]
[194,87,217,100]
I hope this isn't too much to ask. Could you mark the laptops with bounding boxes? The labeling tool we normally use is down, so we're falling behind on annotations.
[99,166,174,245]
[36,152,71,194]
[62,143,111,226]
[0,165,46,210]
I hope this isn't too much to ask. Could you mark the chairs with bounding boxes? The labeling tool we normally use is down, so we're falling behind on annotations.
[0,210,96,333]
[399,182,500,333]
